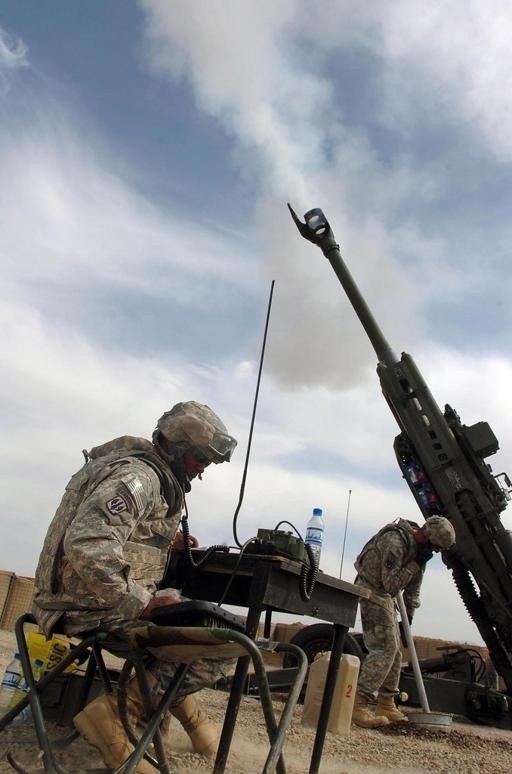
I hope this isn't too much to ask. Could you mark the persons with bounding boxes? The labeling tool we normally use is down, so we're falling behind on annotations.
[353,513,457,729]
[30,399,237,774]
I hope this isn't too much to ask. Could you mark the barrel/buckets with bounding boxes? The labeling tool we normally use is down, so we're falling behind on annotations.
[13,629,72,705]
[301,652,359,737]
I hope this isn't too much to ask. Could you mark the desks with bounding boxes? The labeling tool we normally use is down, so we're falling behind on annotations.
[162,545,370,774]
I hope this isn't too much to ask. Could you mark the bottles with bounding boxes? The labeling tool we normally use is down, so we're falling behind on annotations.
[305,507,325,569]
[0,653,44,716]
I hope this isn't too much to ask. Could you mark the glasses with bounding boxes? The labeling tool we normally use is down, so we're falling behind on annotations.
[209,431,237,462]
[192,448,210,466]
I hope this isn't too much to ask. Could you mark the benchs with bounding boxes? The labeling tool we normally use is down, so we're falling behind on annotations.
[0,600,310,774]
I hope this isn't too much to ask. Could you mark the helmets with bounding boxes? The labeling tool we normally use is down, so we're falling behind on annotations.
[152,401,238,464]
[424,515,456,549]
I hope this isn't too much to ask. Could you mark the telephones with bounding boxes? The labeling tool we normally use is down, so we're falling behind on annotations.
[152,429,191,493]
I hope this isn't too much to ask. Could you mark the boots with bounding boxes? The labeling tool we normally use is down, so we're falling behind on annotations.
[71,667,161,774]
[353,690,408,728]
[169,694,224,758]
